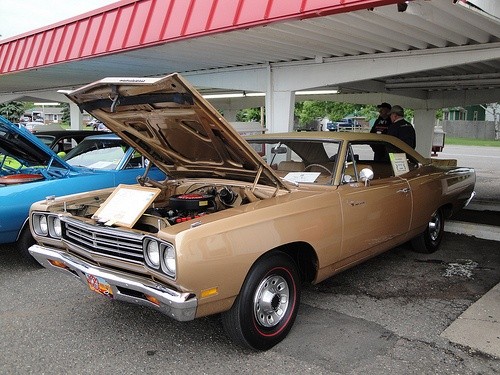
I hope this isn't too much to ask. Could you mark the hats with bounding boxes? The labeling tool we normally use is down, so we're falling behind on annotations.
[386,104,403,115]
[377,102,392,109]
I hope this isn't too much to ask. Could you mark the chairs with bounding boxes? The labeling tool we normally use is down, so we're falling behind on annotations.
[276,161,305,172]
[344,165,372,176]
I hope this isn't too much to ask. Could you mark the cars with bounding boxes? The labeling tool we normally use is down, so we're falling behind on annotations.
[28,71,476,353]
[0,114,170,266]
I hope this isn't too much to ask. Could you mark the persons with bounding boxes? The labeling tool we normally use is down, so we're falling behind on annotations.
[369,103,417,167]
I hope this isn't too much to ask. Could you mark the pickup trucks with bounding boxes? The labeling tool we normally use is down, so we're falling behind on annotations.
[327,117,362,131]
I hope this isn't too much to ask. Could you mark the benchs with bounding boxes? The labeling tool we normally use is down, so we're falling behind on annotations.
[311,163,393,178]
